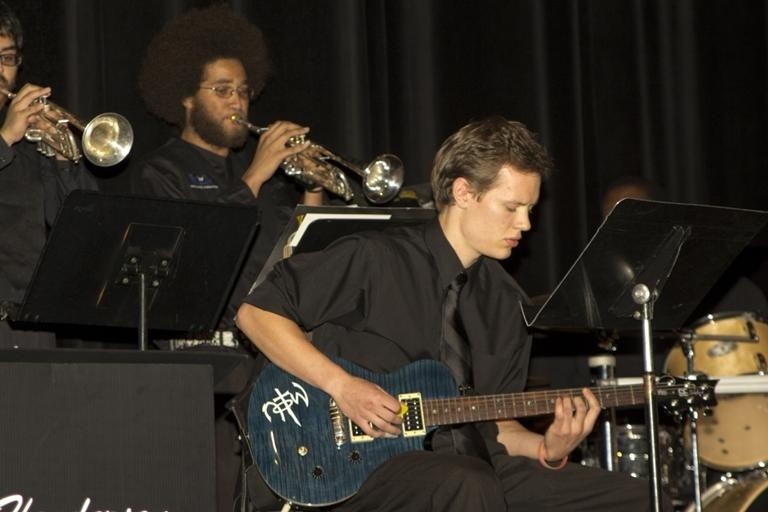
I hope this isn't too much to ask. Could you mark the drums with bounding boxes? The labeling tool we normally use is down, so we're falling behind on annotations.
[663,309,768,471]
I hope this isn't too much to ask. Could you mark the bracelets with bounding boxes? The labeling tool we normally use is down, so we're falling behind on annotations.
[539,440,568,471]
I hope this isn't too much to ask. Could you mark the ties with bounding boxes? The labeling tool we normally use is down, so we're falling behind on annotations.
[441,273,492,467]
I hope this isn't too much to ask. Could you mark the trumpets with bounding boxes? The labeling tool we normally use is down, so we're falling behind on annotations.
[232,110,406,204]
[2,86,134,168]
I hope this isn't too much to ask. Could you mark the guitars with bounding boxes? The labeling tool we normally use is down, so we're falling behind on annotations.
[247,354,719,506]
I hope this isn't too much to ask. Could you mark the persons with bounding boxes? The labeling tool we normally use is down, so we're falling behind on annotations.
[234,112,671,512]
[597,171,768,320]
[0,8,115,347]
[129,7,323,330]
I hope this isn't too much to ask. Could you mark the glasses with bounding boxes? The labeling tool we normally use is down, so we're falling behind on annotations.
[1,53,21,66]
[200,85,251,99]
